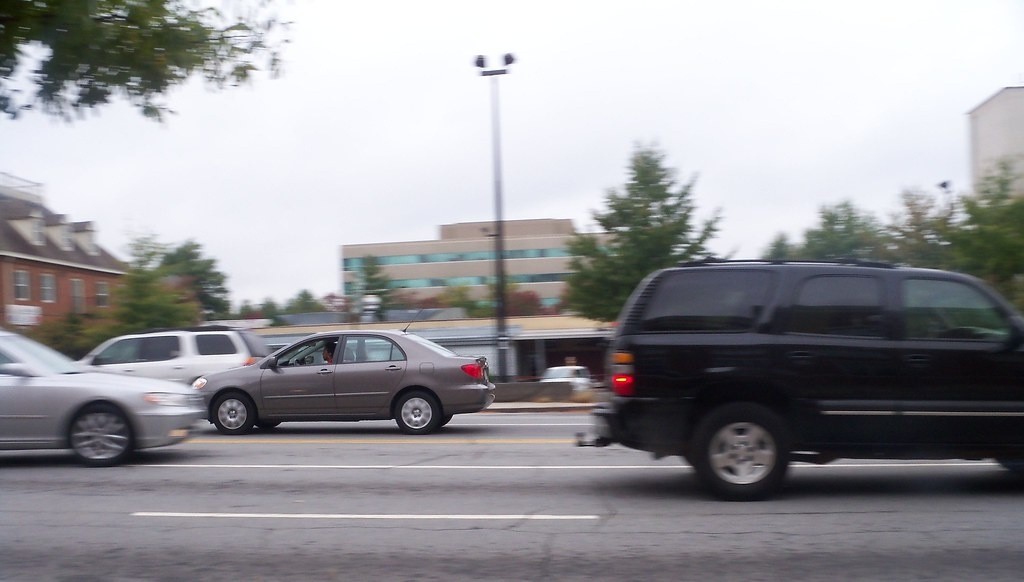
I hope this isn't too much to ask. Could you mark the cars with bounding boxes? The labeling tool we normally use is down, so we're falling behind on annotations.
[190,329,497,435]
[0,329,206,466]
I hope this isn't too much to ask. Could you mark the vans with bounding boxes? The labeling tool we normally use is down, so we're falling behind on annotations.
[539,366,592,391]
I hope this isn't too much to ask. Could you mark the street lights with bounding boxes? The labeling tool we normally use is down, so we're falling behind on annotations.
[475,54,514,383]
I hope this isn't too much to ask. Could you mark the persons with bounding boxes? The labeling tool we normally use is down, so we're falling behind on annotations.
[320,341,337,363]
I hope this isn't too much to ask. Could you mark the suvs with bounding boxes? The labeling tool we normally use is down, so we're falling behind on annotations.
[573,256,1024,500]
[77,325,274,419]
